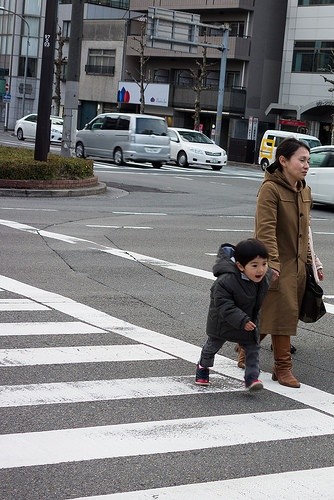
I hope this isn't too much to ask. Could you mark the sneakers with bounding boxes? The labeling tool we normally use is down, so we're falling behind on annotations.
[195,364,209,385]
[245,380,262,392]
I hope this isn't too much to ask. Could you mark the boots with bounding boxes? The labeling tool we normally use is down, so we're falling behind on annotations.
[234,334,267,368]
[271,334,301,388]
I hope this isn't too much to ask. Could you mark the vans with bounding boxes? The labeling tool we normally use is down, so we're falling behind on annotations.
[258,129,326,172]
[74,112,171,169]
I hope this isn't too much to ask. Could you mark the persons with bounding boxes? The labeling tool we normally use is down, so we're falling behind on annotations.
[234,137,314,388]
[269,215,324,354]
[196,237,278,395]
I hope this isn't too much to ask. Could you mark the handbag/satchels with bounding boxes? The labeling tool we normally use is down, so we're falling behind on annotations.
[300,266,326,323]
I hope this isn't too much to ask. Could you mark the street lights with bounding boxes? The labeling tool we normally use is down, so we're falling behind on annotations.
[0,7,31,119]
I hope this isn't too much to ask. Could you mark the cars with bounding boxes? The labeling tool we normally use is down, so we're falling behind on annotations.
[301,145,334,208]
[14,114,79,143]
[167,127,228,171]
